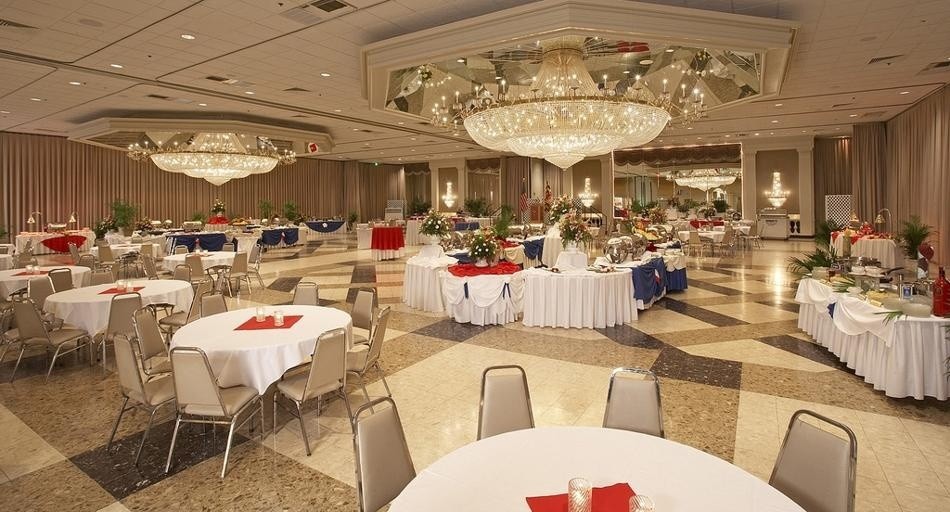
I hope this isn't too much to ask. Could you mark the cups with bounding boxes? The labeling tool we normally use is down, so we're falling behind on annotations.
[627,494,657,511]
[828,270,836,283]
[273,309,284,326]
[568,476,592,511]
[116,280,134,294]
[126,241,208,257]
[25,265,40,275]
[255,305,265,322]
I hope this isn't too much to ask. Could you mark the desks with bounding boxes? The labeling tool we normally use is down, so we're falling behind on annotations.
[404,232,689,330]
[374,427,804,512]
[798,218,950,402]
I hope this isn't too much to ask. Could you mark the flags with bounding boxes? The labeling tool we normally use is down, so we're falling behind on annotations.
[520,184,528,212]
[545,186,553,213]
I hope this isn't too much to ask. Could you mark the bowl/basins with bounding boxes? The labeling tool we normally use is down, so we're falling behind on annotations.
[847,286,863,295]
[852,266,881,274]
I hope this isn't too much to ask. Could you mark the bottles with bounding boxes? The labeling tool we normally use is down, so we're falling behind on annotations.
[904,286,910,296]
[931,266,950,318]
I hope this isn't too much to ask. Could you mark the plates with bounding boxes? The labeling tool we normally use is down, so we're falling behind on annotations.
[883,297,932,319]
[811,267,829,280]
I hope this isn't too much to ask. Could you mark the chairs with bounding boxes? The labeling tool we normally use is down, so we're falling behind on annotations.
[352,395,417,511]
[0,205,264,384]
[105,281,394,479]
[601,367,665,441]
[475,366,534,441]
[768,410,858,512]
[267,207,763,257]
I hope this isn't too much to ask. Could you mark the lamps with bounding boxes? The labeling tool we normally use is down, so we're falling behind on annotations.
[579,178,599,208]
[673,166,737,194]
[427,34,712,171]
[441,180,458,208]
[761,168,792,208]
[123,127,298,191]
